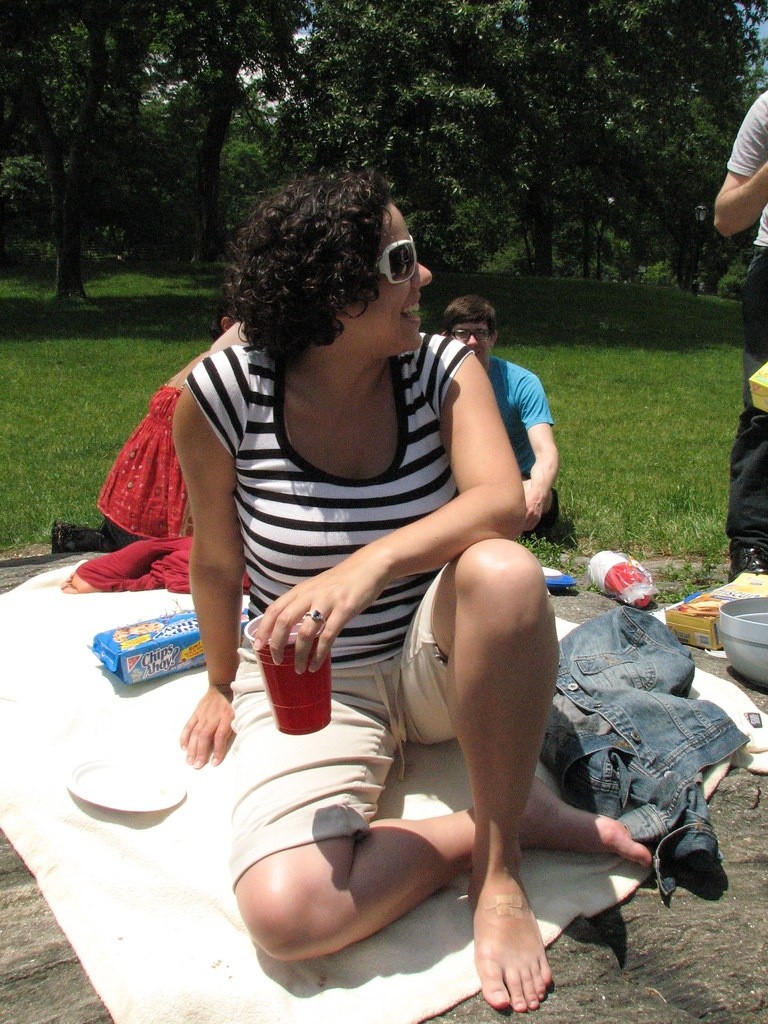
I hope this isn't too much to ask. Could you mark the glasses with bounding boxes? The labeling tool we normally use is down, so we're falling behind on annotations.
[449,329,490,342]
[376,235,418,285]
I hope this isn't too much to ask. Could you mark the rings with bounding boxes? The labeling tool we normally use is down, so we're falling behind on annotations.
[306,610,326,623]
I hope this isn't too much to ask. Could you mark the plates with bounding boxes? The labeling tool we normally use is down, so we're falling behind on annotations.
[67,750,187,813]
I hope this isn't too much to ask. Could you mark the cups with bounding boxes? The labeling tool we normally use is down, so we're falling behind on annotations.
[243,610,332,736]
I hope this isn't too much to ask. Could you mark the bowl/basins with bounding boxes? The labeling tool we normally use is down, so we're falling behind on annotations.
[718,597,768,690]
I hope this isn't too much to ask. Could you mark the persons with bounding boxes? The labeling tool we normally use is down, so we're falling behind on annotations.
[50,282,579,595]
[169,173,654,1013]
[714,90,768,582]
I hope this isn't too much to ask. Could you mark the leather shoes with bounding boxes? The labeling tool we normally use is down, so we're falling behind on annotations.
[727,547,768,582]
